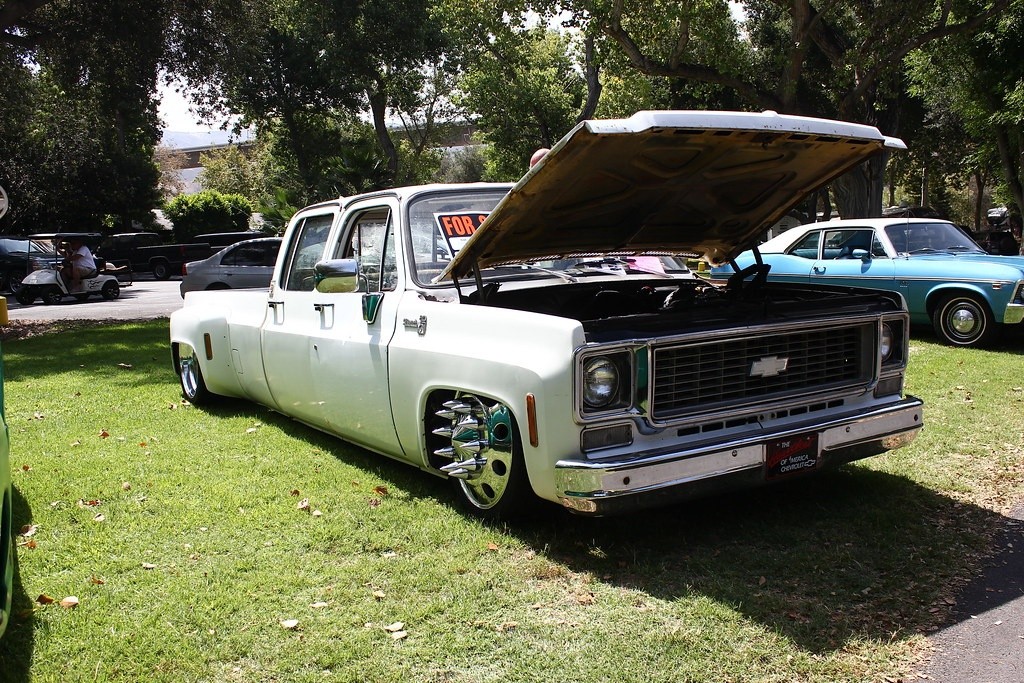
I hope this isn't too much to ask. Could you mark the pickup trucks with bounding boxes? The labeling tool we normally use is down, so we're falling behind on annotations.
[95,233,213,281]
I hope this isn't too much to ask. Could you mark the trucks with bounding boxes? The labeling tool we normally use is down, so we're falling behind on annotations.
[169,109,925,525]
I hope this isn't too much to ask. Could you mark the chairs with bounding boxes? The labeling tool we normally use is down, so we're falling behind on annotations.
[838,244,868,260]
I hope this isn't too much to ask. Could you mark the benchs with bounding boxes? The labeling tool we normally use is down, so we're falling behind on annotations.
[793,249,842,259]
[359,269,443,293]
[81,257,106,279]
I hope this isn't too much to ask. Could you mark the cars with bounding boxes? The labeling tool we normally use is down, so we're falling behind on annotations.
[192,231,270,258]
[709,217,1024,349]
[0,235,65,296]
[179,237,284,299]
[659,256,696,279]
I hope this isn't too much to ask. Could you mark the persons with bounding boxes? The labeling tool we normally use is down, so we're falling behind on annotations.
[528,148,550,170]
[56,238,97,292]
